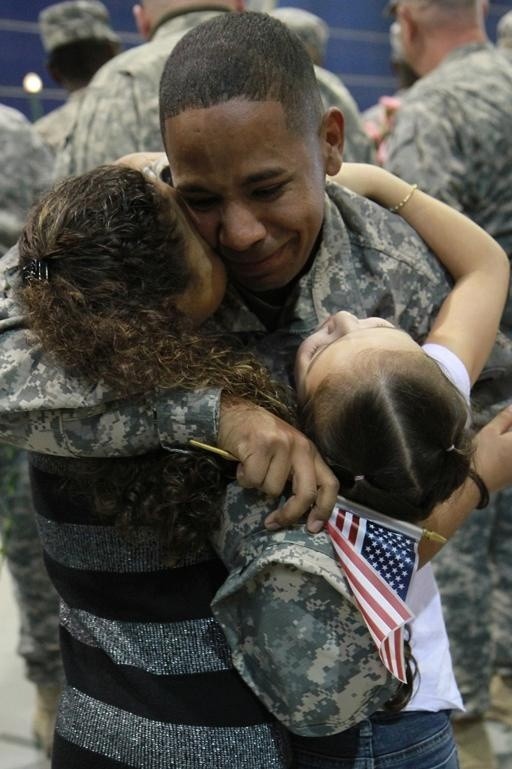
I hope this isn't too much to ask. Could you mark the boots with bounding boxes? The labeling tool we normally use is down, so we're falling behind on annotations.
[483,671,510,724]
[32,680,61,756]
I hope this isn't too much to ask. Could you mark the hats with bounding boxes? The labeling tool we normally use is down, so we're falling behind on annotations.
[271,7,326,63]
[38,0,123,57]
[207,482,398,740]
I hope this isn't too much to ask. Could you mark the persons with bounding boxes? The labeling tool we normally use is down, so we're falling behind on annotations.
[0,0,512,768]
